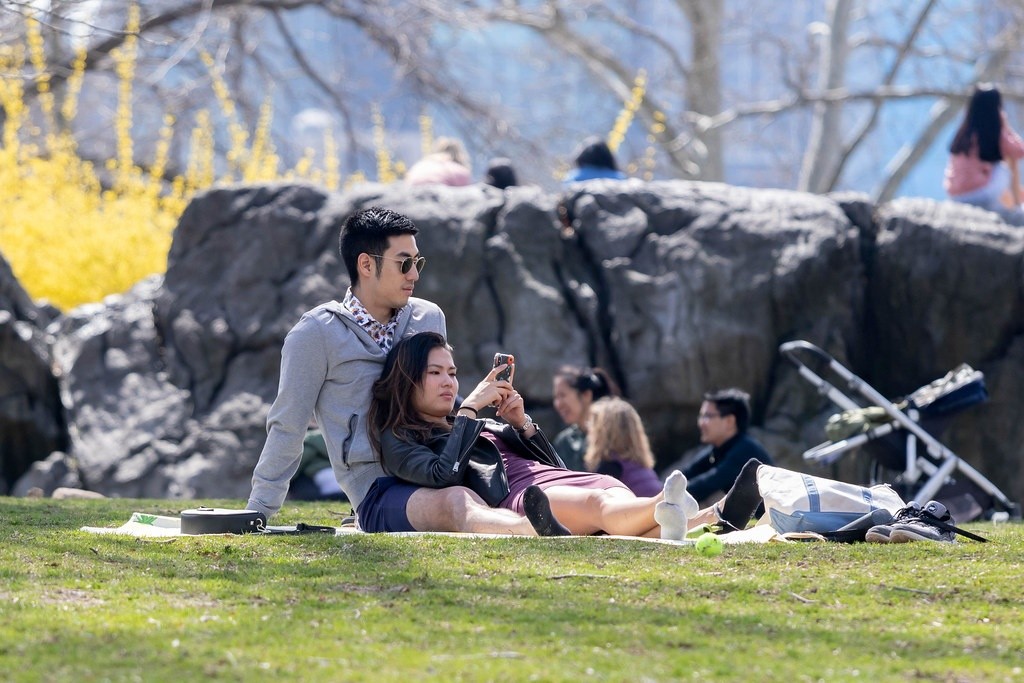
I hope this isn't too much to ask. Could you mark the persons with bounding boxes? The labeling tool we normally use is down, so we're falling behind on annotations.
[549,364,623,473]
[481,157,519,189]
[580,394,663,498]
[561,134,629,186]
[403,134,473,188]
[246,207,769,537]
[370,330,699,541]
[945,82,1024,228]
[673,388,776,527]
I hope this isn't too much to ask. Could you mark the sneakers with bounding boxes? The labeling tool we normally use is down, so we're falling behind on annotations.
[866,501,957,544]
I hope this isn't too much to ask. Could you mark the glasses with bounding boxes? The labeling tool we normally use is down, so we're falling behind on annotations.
[369,254,426,275]
[697,413,719,423]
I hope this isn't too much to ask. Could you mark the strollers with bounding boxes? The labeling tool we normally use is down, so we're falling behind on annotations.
[778,341,1020,522]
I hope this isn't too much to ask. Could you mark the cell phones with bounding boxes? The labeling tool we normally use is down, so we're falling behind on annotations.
[488,352,515,408]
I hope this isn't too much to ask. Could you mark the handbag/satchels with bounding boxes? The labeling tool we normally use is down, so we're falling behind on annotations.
[757,464,991,543]
[181,506,335,535]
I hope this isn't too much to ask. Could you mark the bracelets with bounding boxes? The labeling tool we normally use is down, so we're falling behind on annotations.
[457,406,478,415]
[516,412,532,433]
